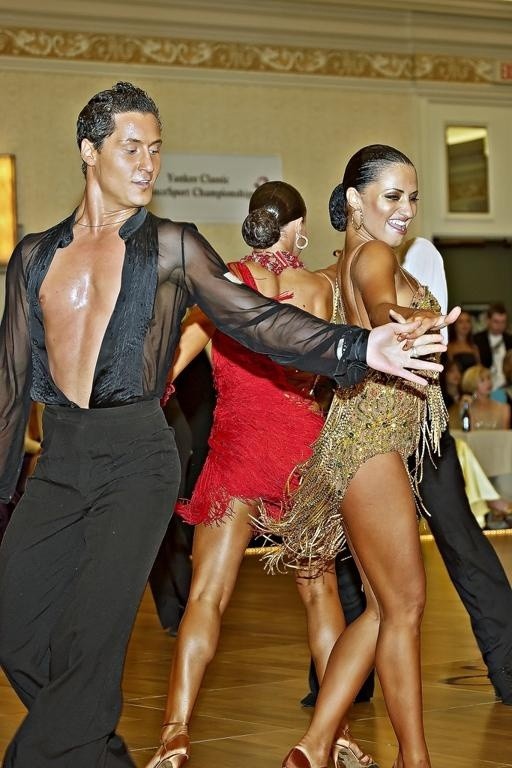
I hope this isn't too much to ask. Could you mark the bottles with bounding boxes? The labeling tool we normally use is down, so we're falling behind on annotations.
[462,402,471,431]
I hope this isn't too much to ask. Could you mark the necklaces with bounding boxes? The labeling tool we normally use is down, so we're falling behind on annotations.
[75,220,129,228]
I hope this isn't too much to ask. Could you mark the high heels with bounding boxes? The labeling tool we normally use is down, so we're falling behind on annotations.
[330,727,380,768]
[493,502,511,516]
[145,721,191,768]
[282,745,311,768]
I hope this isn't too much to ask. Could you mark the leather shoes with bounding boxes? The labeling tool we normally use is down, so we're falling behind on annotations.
[300,690,318,710]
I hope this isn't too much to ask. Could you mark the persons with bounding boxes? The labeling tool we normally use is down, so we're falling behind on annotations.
[283,141,450,768]
[452,437,512,527]
[144,179,383,768]
[299,236,511,707]
[0,81,449,768]
[150,322,218,634]
[444,300,512,430]
